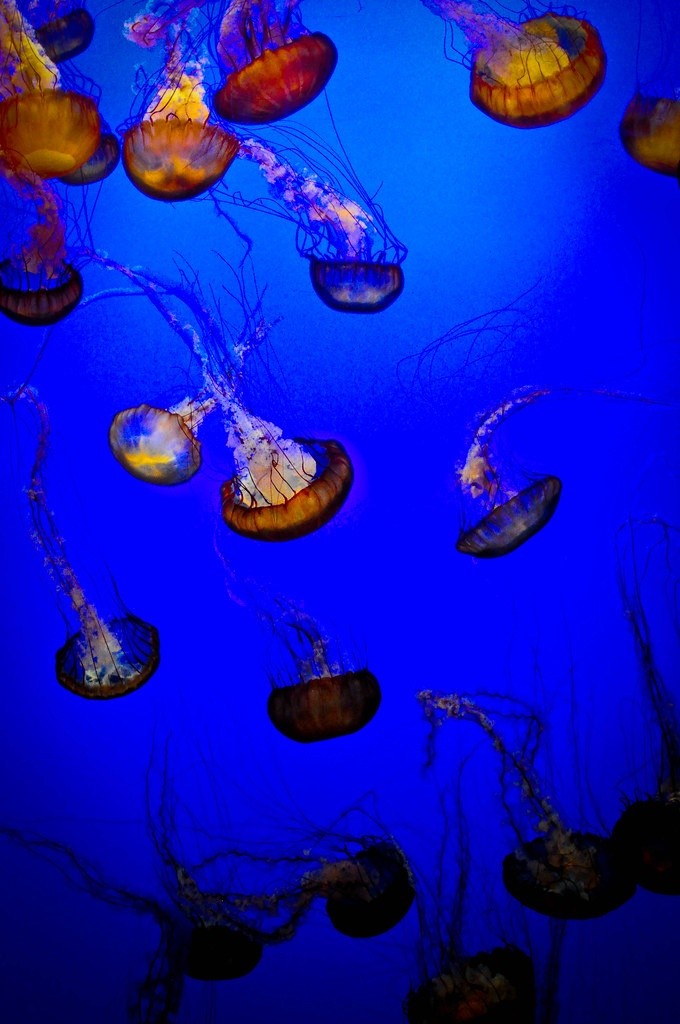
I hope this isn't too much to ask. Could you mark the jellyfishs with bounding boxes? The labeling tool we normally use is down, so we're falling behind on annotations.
[0,0,680,1024]
